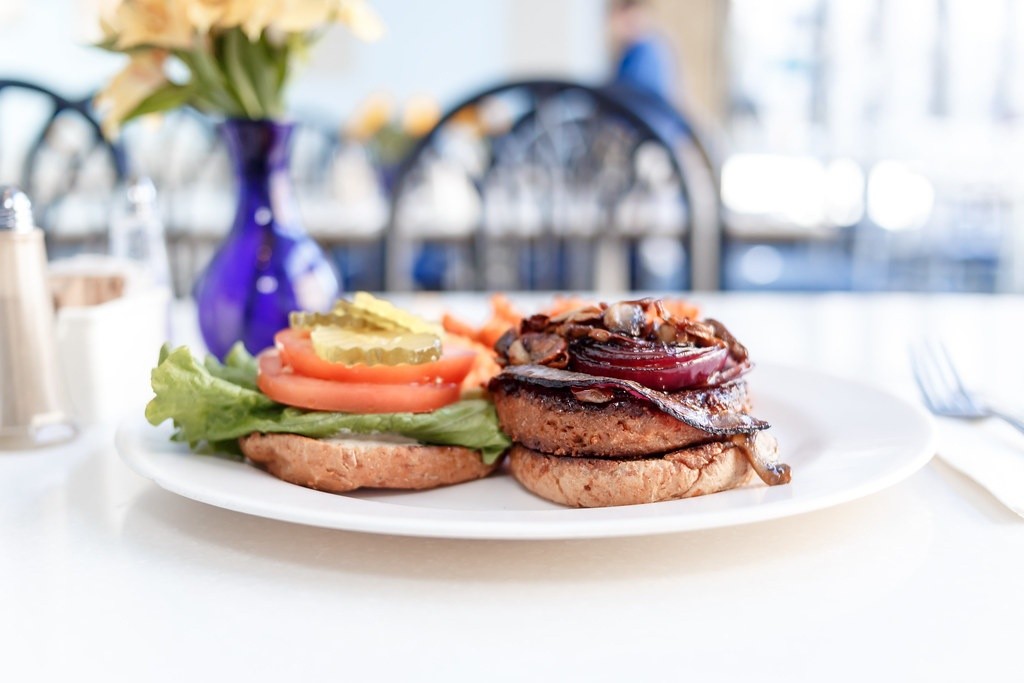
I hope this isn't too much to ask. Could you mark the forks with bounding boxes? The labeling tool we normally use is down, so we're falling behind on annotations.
[909,343,1024,434]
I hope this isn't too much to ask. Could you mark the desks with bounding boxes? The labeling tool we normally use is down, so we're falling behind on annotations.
[32,177,846,293]
[0,289,1024,683]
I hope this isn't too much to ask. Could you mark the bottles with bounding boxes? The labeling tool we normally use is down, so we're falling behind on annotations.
[0,186,72,452]
[110,179,175,331]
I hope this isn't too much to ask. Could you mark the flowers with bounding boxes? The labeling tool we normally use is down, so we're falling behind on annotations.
[85,0,390,147]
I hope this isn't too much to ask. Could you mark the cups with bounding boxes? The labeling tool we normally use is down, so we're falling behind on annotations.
[50,255,137,377]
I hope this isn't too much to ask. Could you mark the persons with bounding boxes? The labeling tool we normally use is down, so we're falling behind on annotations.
[594,0,673,139]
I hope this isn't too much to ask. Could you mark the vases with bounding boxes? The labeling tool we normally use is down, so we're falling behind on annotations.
[192,115,342,365]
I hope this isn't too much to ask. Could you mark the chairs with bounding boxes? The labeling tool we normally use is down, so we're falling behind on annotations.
[379,75,696,293]
[1,74,131,246]
[470,81,729,292]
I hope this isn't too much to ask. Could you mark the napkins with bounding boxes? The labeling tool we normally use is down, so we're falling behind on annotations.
[910,381,1024,519]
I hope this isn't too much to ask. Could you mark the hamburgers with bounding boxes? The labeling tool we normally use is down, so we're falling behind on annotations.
[146,288,507,489]
[493,299,779,508]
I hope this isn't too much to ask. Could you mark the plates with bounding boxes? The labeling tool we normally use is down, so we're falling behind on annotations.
[115,371,937,539]
